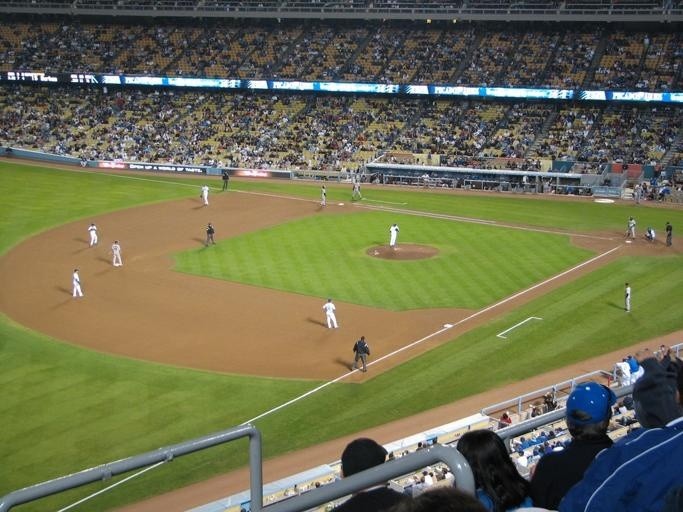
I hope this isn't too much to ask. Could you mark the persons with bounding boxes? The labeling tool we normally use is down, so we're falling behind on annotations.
[283,336,683,512]
[0,19,683,206]
[206,221,216,247]
[666,222,672,247]
[645,226,656,242]
[624,215,636,239]
[72,269,84,297]
[111,240,123,267]
[87,223,98,246]
[389,223,399,247]
[322,299,339,329]
[625,283,631,312]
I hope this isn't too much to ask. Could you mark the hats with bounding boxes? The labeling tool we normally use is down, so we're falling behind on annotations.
[565,380,620,427]
